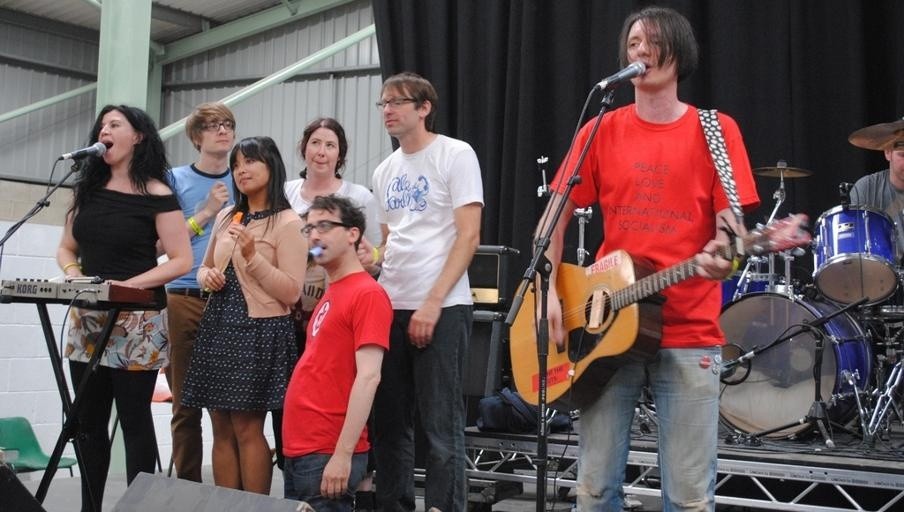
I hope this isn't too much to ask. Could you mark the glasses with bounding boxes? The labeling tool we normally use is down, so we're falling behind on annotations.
[299,220,354,238]
[375,96,423,109]
[207,118,235,131]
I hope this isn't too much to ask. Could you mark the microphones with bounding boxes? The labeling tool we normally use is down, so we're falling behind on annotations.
[593,61,646,91]
[719,359,737,380]
[58,141,107,160]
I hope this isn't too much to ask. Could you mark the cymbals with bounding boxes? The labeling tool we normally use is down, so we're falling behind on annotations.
[752,166,809,179]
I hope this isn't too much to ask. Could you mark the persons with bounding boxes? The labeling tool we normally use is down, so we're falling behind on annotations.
[279,197,396,510]
[354,71,484,511]
[57,104,194,510]
[276,119,385,342]
[154,104,243,482]
[841,120,903,275]
[193,134,309,496]
[529,9,759,511]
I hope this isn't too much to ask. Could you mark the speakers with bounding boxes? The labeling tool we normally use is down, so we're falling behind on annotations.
[467,244,522,309]
[110,470,317,511]
[462,310,513,427]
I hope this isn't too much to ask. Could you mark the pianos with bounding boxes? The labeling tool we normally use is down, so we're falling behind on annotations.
[0,278,154,304]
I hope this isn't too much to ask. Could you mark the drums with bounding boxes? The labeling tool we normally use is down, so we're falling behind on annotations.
[811,206,900,308]
[723,272,778,306]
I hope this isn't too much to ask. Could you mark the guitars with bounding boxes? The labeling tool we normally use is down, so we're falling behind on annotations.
[510,214,811,407]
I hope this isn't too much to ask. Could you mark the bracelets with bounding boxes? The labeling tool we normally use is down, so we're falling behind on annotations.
[186,215,202,235]
[372,246,379,266]
[61,261,79,272]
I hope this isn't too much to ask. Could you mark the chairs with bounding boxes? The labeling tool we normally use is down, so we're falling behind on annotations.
[0,418,79,478]
